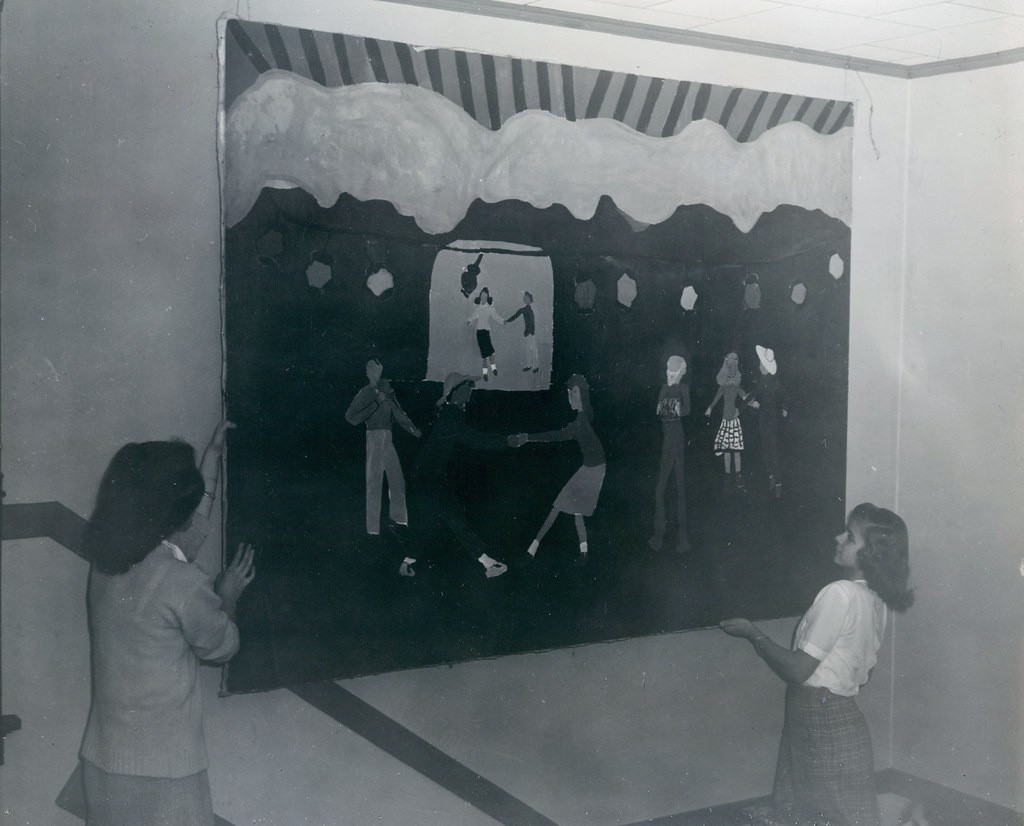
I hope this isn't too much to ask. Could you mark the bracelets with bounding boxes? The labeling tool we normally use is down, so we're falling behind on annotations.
[203,491,216,500]
[202,474,217,484]
[755,635,768,658]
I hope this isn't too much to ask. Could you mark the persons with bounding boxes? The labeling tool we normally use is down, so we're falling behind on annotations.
[717,503,915,826]
[55,418,256,826]
